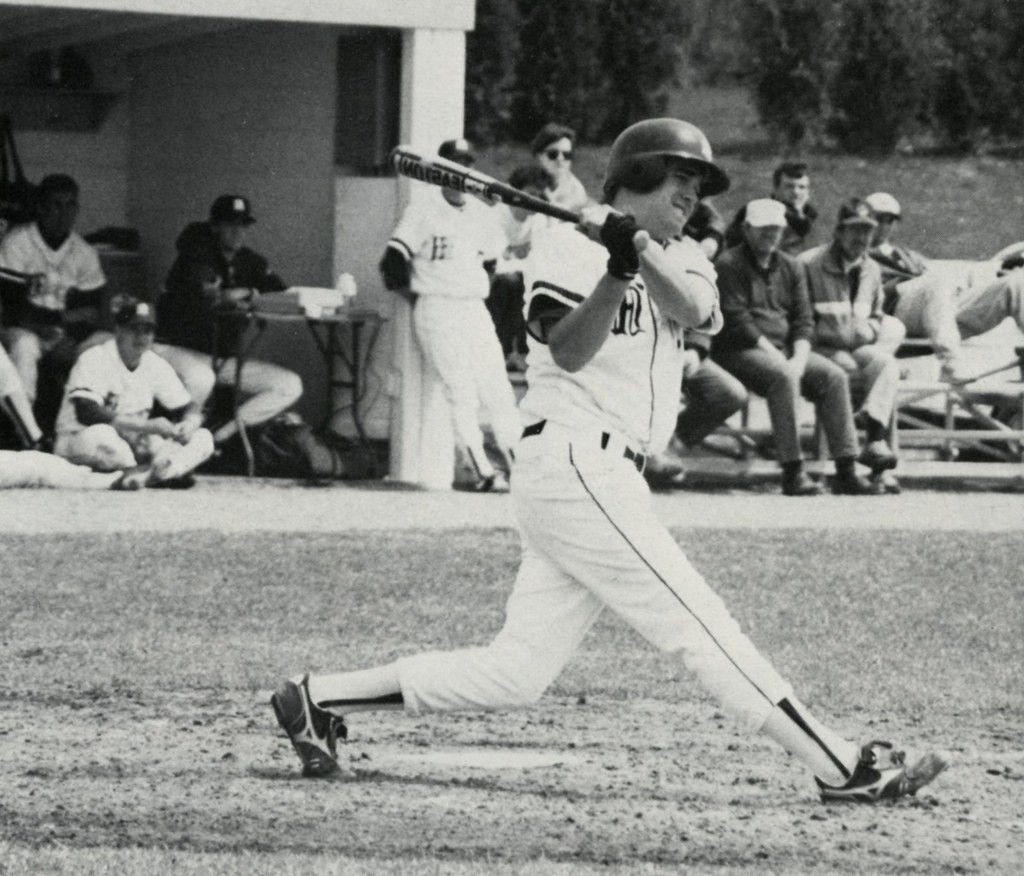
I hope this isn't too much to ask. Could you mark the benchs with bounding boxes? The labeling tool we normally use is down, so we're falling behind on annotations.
[507,338,1024,493]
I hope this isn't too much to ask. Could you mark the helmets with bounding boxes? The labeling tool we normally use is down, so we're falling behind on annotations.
[603,117,730,198]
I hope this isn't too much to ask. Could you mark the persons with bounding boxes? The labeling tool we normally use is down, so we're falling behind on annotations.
[267,117,949,806]
[481,120,588,369]
[377,136,525,493]
[674,163,1024,496]
[0,171,341,489]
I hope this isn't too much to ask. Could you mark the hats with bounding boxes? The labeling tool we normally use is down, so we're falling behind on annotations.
[837,198,879,227]
[745,199,787,228]
[118,299,157,327]
[867,193,902,214]
[440,138,476,160]
[212,196,255,223]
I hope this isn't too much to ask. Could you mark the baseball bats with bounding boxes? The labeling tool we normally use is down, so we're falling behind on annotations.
[387,143,651,252]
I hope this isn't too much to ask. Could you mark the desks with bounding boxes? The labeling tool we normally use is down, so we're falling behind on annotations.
[209,310,391,482]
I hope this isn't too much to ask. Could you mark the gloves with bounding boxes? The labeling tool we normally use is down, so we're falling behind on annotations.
[600,212,640,281]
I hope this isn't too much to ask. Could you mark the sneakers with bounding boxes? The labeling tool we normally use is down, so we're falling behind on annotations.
[814,741,946,808]
[271,674,347,778]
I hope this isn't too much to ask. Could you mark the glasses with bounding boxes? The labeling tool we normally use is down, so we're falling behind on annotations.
[547,150,573,160]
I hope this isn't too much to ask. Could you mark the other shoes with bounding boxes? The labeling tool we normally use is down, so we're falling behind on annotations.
[159,476,194,488]
[869,475,900,494]
[832,475,873,494]
[781,471,824,495]
[455,473,509,493]
